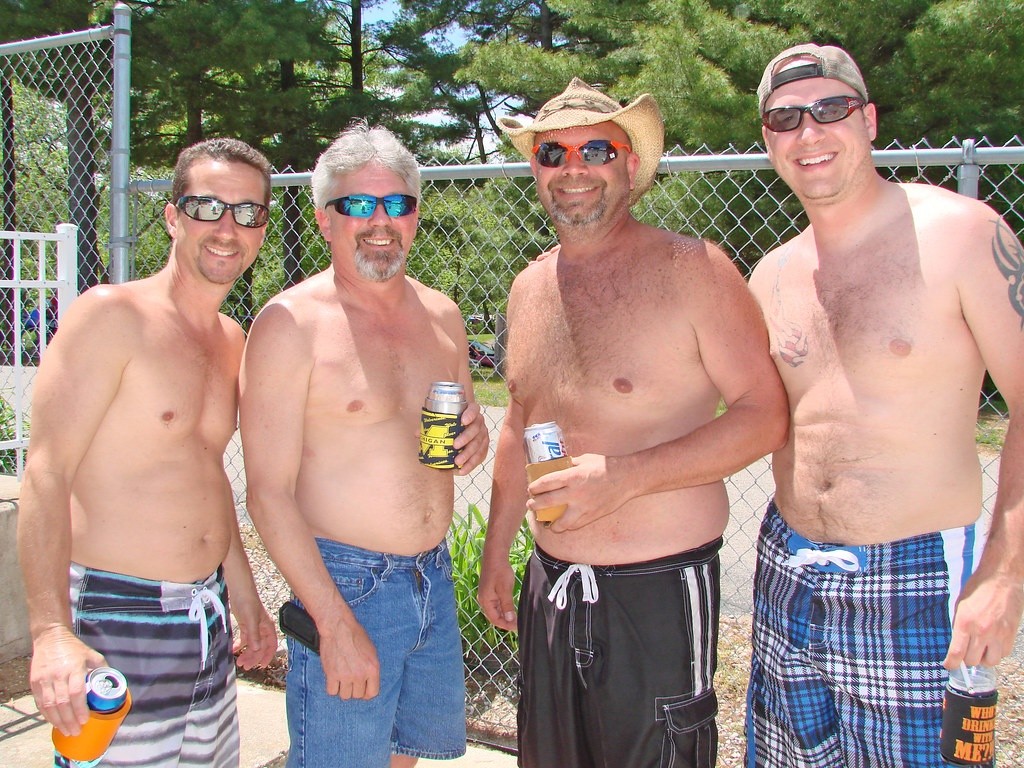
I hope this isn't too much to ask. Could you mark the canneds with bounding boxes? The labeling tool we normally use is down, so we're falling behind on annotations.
[523,421,568,465]
[84,667,128,714]
[427,381,466,402]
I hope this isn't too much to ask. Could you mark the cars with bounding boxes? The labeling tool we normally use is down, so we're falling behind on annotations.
[468,340,495,368]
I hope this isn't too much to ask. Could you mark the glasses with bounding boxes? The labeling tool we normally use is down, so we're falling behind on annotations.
[324,193,417,218]
[760,95,866,132]
[531,139,631,168]
[175,196,269,228]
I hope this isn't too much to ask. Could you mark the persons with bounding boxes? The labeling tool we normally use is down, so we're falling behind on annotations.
[472,75,793,768]
[237,119,491,767]
[15,136,281,768]
[522,43,1024,768]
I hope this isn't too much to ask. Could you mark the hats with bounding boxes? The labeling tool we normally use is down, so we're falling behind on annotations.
[757,43,869,118]
[495,77,665,207]
